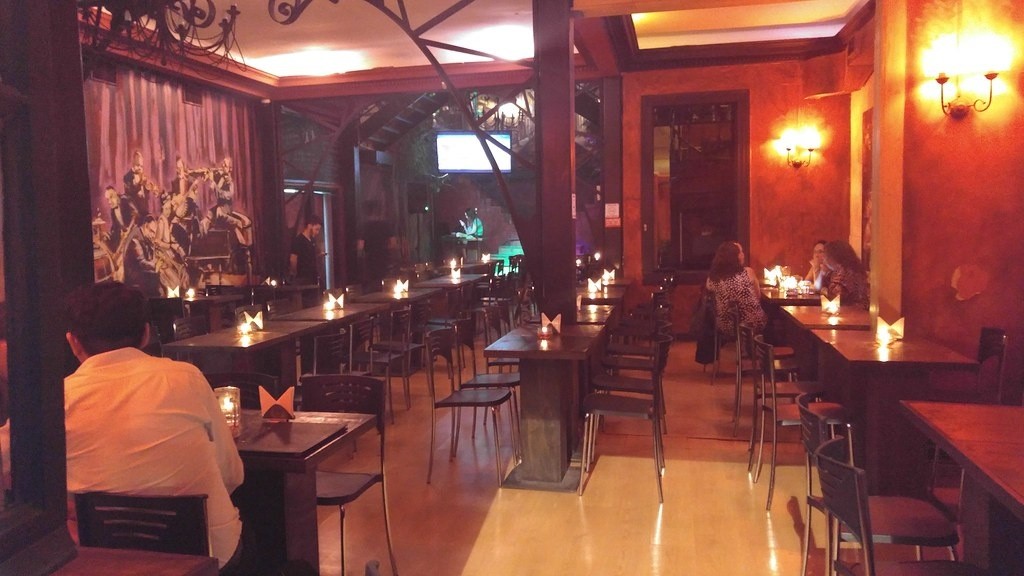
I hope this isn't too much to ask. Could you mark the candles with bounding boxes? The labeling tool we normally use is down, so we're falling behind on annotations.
[537,326,553,340]
[213,386,242,427]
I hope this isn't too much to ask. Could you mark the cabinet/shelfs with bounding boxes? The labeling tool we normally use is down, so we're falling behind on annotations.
[413,271,489,348]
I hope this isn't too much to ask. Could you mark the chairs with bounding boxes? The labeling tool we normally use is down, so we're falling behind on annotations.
[687,286,1009,576]
[73,491,210,558]
[577,272,681,503]
[299,372,398,576]
[147,255,538,490]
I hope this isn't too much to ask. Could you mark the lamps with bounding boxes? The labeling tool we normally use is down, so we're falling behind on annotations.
[77,0,247,92]
[786,147,814,170]
[935,68,1000,121]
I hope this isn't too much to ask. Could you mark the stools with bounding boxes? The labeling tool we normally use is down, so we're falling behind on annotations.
[466,238,483,261]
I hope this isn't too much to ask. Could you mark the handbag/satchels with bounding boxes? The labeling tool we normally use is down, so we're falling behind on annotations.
[696,291,720,364]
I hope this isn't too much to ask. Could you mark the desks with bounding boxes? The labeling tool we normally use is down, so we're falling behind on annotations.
[161,318,329,409]
[235,409,378,576]
[278,284,320,310]
[52,547,220,576]
[434,257,498,277]
[272,302,392,370]
[778,304,878,426]
[577,286,625,376]
[761,287,825,347]
[348,286,443,377]
[897,399,1024,576]
[182,294,246,333]
[808,328,981,490]
[577,276,634,322]
[484,322,606,495]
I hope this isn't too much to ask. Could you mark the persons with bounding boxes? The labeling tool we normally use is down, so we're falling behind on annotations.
[456,207,485,261]
[706,239,768,343]
[356,212,401,294]
[287,214,325,284]
[0,279,259,576]
[804,240,870,310]
